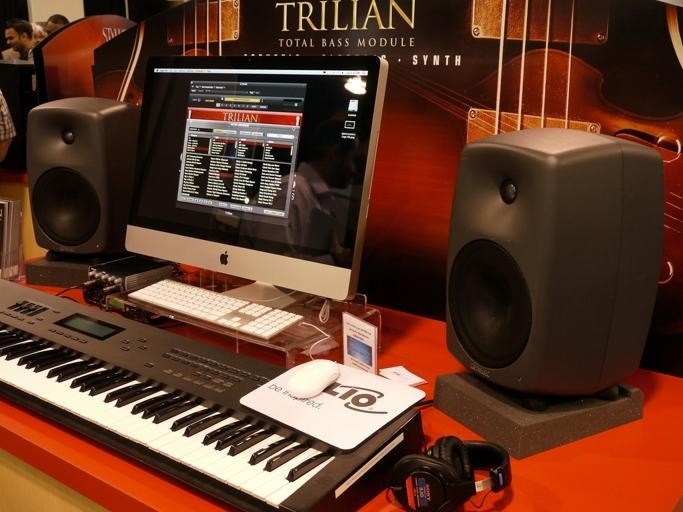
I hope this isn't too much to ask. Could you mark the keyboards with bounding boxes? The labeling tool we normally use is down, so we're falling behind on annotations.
[128,279,305,341]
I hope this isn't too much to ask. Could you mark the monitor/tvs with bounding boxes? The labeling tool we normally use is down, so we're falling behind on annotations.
[124,55,389,309]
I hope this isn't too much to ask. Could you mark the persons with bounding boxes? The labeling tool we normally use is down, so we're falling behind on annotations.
[0,17,69,163]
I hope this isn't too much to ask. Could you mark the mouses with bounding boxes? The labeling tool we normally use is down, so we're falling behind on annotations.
[287,359,340,399]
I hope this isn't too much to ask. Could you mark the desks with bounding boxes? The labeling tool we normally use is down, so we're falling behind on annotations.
[0,255,683,512]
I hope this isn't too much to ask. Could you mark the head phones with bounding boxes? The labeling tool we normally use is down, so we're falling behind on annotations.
[385,435,511,512]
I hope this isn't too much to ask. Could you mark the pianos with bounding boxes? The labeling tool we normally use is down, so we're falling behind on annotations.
[1,279,423,511]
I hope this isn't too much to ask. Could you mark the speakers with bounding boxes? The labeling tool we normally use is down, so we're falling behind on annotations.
[25,97,141,254]
[447,128,663,398]
